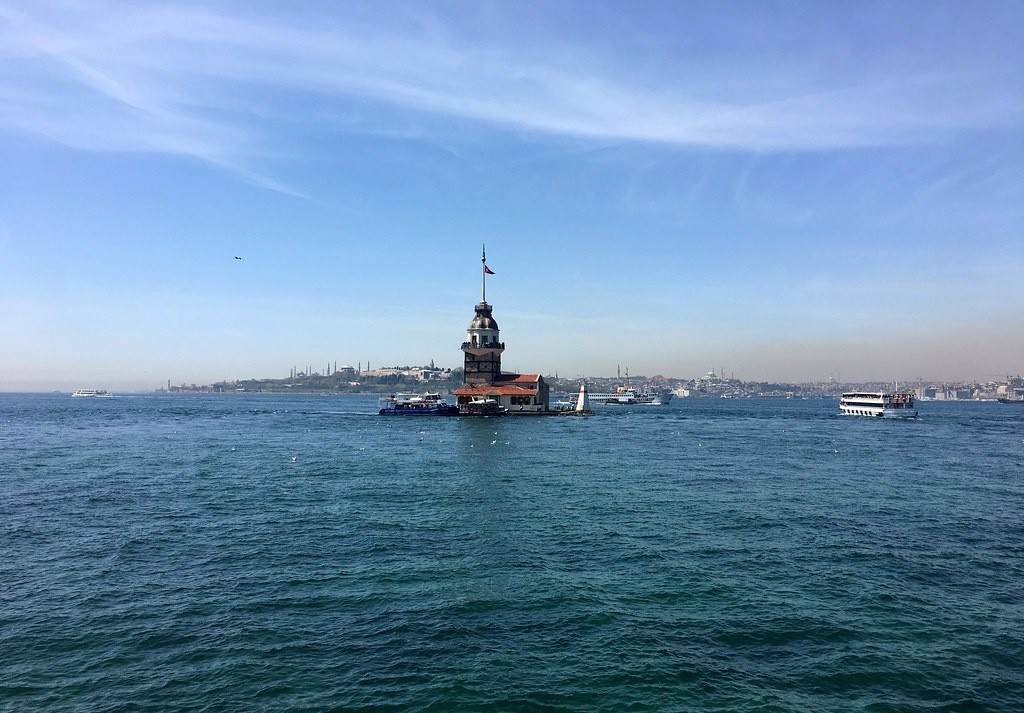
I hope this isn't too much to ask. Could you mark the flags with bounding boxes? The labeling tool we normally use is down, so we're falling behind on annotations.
[485,265,495,274]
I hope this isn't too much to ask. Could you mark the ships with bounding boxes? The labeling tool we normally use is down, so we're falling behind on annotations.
[567,385,673,406]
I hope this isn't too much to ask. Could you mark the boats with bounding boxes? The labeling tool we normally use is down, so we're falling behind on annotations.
[71,389,119,400]
[839,392,918,419]
[374,391,508,419]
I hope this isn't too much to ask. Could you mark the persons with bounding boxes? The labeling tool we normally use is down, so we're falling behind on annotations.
[470,403,499,414]
[843,394,905,402]
[553,405,576,411]
